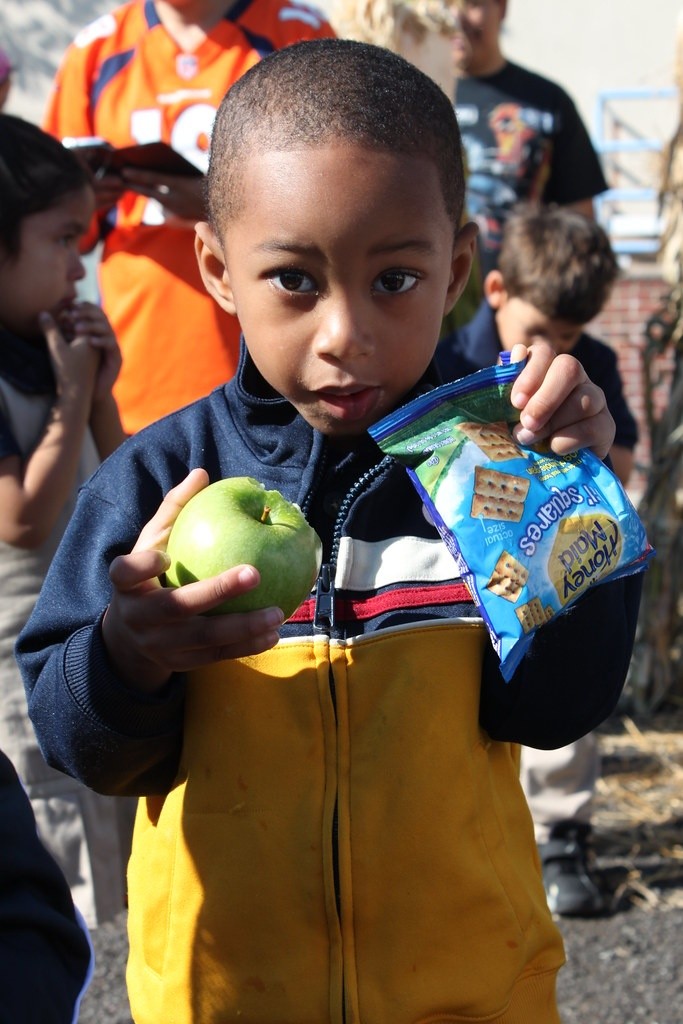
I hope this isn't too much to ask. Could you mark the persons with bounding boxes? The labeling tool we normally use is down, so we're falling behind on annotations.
[433,211,639,917]
[0,113,127,893]
[329,1,485,340]
[40,0,342,440]
[16,40,639,1024]
[440,0,611,303]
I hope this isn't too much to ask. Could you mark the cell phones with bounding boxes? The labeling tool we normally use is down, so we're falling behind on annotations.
[65,137,116,176]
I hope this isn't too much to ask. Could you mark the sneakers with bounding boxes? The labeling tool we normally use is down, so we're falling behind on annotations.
[533,815,612,920]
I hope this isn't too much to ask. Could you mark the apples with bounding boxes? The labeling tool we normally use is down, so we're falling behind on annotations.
[160,476,322,627]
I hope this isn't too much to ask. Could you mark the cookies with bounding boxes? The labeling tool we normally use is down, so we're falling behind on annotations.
[455,420,556,634]
